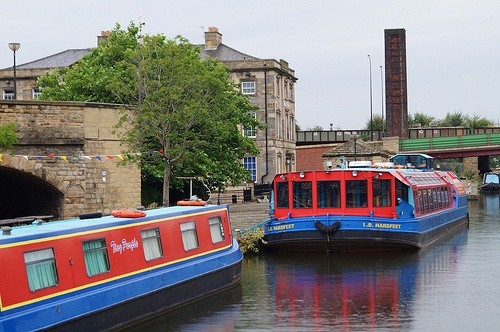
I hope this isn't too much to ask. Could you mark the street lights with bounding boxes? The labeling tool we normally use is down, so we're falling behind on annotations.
[379,66,384,121]
[8,42,21,100]
[367,55,373,130]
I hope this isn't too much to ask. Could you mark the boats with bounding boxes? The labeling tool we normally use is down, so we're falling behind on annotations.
[0,180,244,332]
[256,160,470,250]
[479,172,500,194]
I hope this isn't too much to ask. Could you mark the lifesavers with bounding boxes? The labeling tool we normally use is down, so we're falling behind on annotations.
[177,200,206,206]
[112,209,146,218]
[79,213,102,219]
